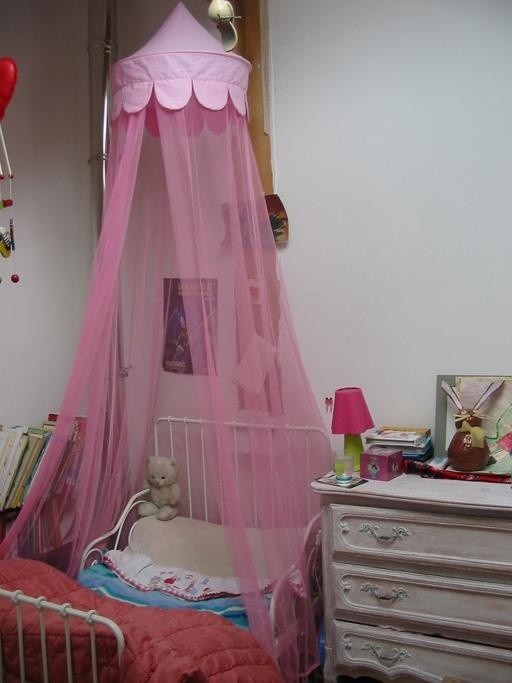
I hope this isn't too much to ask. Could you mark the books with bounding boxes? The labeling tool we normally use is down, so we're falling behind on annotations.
[365,424,433,462]
[4,498,77,559]
[0,412,88,511]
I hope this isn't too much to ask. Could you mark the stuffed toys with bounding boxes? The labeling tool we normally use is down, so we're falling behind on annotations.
[137,455,181,521]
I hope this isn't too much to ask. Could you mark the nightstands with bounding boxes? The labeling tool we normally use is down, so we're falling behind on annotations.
[311,463,512,683]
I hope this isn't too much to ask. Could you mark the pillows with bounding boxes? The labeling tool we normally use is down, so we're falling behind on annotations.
[126,511,309,586]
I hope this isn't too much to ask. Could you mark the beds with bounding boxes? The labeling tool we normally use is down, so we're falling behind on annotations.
[1,418,333,683]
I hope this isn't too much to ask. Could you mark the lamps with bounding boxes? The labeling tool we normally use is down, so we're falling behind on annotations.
[331,386,375,471]
